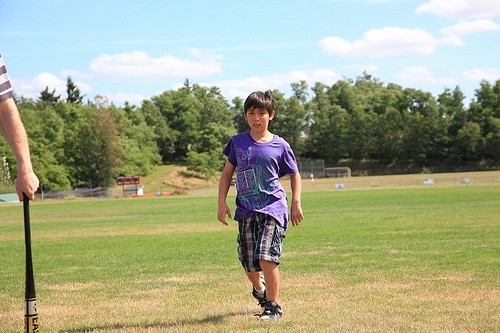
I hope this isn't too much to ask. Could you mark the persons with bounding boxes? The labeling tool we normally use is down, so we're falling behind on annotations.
[0,54,41,203]
[214,90,304,322]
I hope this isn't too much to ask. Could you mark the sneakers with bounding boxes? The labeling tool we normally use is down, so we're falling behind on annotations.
[251,275,282,321]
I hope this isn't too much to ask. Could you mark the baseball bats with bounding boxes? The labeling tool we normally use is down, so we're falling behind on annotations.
[19,191,39,333]
